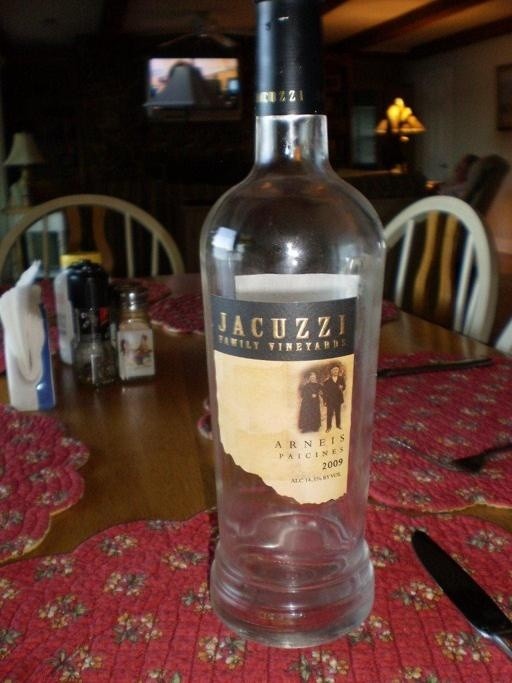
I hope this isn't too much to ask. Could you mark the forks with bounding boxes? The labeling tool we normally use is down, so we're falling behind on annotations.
[392,435,509,476]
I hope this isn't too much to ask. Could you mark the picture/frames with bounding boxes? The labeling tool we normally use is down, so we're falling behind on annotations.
[496,63,512,131]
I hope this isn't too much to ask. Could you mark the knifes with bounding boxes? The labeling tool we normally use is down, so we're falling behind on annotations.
[413,529,512,656]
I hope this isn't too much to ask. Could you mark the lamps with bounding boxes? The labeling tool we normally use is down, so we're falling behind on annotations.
[4,131,44,207]
[375,100,425,173]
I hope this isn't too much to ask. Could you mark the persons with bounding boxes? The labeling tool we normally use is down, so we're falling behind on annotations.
[134,335,149,366]
[298,371,327,433]
[431,154,480,198]
[119,340,132,360]
[323,365,346,432]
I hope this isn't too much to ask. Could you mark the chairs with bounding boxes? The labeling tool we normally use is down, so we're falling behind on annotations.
[0,192,185,278]
[497,314,512,362]
[382,193,498,345]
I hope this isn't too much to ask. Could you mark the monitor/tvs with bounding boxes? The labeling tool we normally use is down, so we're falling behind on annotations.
[148,57,242,122]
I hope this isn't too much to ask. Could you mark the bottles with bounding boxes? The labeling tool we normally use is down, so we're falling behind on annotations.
[196,0,380,651]
[51,246,154,387]
